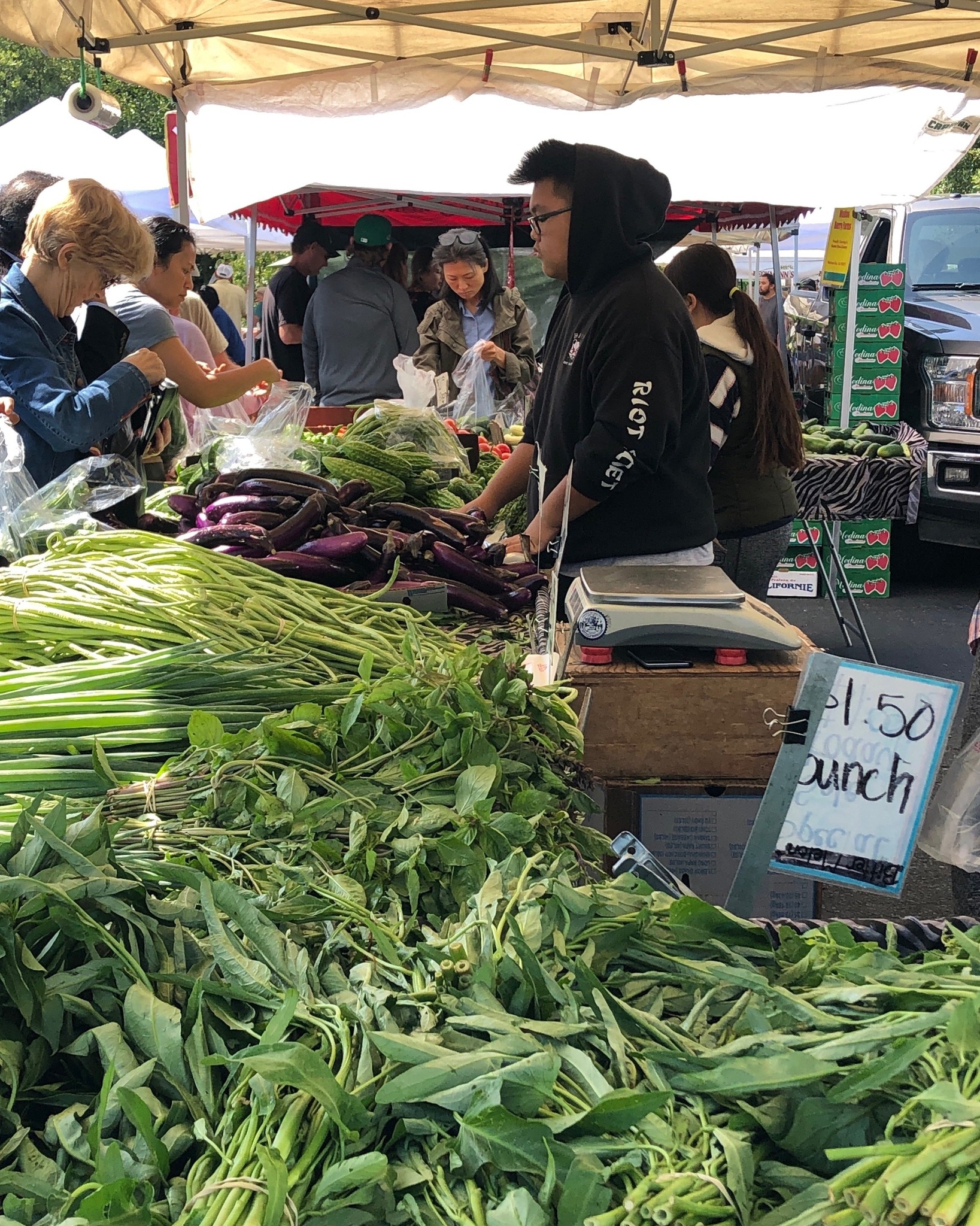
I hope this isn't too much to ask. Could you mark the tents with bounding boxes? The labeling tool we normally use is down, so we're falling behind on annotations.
[229,179,821,397]
[1,0,980,365]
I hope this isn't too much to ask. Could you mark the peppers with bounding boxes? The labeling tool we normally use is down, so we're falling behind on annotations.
[286,424,345,447]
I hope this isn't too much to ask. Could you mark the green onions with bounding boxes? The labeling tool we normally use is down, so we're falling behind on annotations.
[0,634,362,807]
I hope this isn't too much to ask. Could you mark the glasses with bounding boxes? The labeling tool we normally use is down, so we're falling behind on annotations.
[97,265,115,291]
[527,207,572,234]
[437,230,480,247]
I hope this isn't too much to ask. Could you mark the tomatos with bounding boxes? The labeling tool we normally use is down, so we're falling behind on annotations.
[442,418,512,460]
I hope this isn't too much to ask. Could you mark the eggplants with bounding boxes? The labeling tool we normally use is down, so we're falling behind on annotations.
[101,465,548,621]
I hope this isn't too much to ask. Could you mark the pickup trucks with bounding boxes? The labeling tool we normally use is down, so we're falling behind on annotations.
[789,191,980,585]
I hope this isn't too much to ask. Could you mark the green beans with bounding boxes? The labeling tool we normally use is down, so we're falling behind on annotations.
[0,526,472,684]
[334,402,464,457]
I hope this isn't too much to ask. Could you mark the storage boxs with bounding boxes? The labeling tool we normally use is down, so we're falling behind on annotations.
[766,518,890,597]
[825,263,906,427]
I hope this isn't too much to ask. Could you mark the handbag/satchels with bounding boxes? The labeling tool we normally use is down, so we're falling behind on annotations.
[129,376,188,460]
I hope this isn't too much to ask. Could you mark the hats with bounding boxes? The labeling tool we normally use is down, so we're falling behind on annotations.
[353,213,399,247]
[216,263,234,278]
[198,285,220,310]
[292,222,341,258]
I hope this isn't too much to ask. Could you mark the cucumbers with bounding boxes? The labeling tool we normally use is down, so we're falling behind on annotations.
[799,417,905,458]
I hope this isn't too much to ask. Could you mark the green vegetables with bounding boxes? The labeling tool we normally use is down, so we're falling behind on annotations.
[83,642,606,821]
[1,792,980,1226]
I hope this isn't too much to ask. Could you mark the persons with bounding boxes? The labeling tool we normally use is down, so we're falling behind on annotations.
[1,171,283,489]
[663,241,806,602]
[261,214,536,407]
[453,138,716,623]
[758,272,783,350]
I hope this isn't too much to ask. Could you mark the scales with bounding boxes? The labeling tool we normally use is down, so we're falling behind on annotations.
[564,564,802,665]
